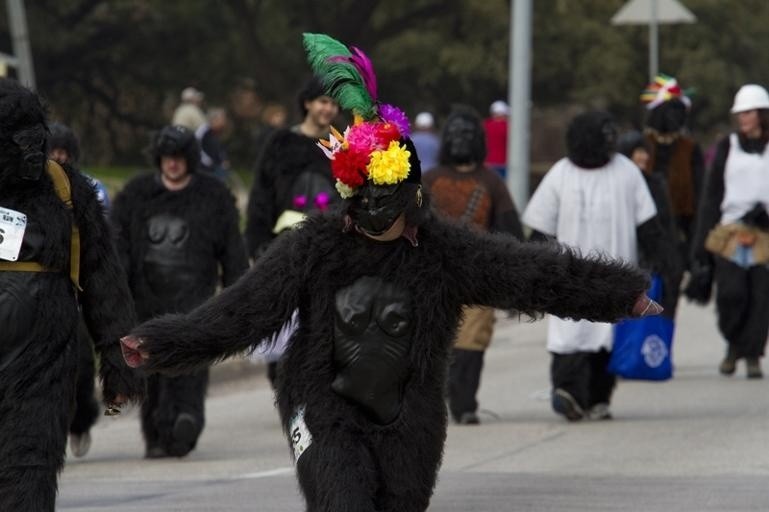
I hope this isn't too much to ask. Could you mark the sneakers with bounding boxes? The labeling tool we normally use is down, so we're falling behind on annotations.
[71,429,92,458]
[720,353,741,376]
[552,389,583,422]
[457,408,477,425]
[590,400,612,421]
[746,356,763,378]
[146,443,168,460]
[166,413,200,456]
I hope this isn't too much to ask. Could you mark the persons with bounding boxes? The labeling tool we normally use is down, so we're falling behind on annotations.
[41,121,110,222]
[638,71,706,318]
[242,74,352,397]
[0,79,143,511]
[615,131,678,380]
[691,84,769,378]
[119,32,665,512]
[520,110,660,422]
[419,110,524,428]
[108,124,250,461]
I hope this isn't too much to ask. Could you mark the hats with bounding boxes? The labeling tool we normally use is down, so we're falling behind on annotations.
[490,100,511,117]
[729,83,769,113]
[414,112,434,131]
